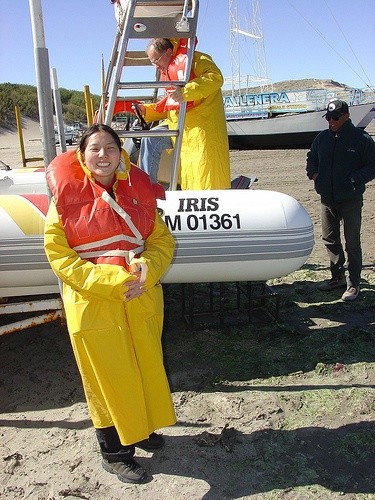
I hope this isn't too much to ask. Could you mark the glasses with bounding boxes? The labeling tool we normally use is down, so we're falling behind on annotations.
[325,113,346,121]
[151,53,165,67]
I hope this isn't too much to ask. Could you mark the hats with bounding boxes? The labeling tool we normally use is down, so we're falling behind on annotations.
[322,100,349,118]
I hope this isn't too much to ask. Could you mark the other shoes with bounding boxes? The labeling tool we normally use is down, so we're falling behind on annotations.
[101,456,148,484]
[318,274,347,291]
[342,286,360,301]
[134,433,165,451]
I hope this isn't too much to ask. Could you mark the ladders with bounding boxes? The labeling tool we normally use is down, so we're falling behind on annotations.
[97,0,199,192]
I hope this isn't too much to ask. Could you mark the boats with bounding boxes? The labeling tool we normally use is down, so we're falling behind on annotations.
[223,88,375,150]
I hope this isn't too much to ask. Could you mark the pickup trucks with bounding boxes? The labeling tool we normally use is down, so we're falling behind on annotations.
[52,125,73,146]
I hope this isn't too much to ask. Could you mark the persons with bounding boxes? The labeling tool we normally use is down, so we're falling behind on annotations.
[131,37,230,190]
[42,122,176,484]
[306,101,374,301]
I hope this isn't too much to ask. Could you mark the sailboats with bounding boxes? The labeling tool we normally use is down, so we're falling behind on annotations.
[0,0,315,299]
